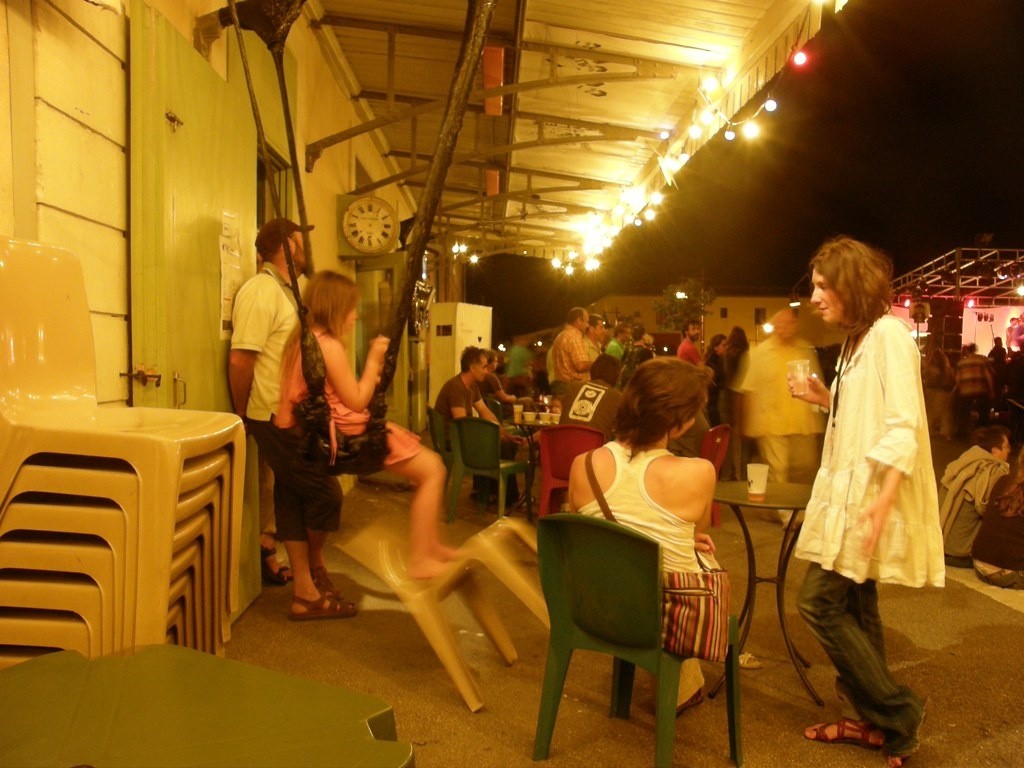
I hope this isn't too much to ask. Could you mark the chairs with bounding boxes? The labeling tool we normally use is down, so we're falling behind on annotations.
[329,512,550,713]
[696,418,735,524]
[446,417,530,521]
[426,403,454,514]
[533,423,608,518]
[528,509,744,768]
[1,235,251,666]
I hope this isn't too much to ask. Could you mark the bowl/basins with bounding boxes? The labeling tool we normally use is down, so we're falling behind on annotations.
[539,413,552,422]
[523,412,537,421]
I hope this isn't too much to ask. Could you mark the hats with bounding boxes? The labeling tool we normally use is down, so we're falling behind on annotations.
[255,217,314,257]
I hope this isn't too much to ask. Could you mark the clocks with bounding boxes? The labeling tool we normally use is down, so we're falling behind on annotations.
[341,198,401,253]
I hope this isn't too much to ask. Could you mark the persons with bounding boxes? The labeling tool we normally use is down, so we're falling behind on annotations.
[434,333,541,517]
[939,425,1024,590]
[274,268,465,580]
[953,336,1024,443]
[260,508,294,587]
[567,358,762,718]
[226,216,358,622]
[547,307,829,532]
[785,235,944,768]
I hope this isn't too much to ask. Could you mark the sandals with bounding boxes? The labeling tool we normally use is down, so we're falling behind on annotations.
[259,537,294,587]
[802,697,932,768]
[285,566,357,621]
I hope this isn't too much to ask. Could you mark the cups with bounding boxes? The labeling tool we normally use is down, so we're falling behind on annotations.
[552,414,560,422]
[512,405,523,423]
[788,360,810,395]
[747,463,768,501]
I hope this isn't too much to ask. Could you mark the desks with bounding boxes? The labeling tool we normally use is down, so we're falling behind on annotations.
[503,415,570,518]
[0,644,412,767]
[690,481,824,708]
